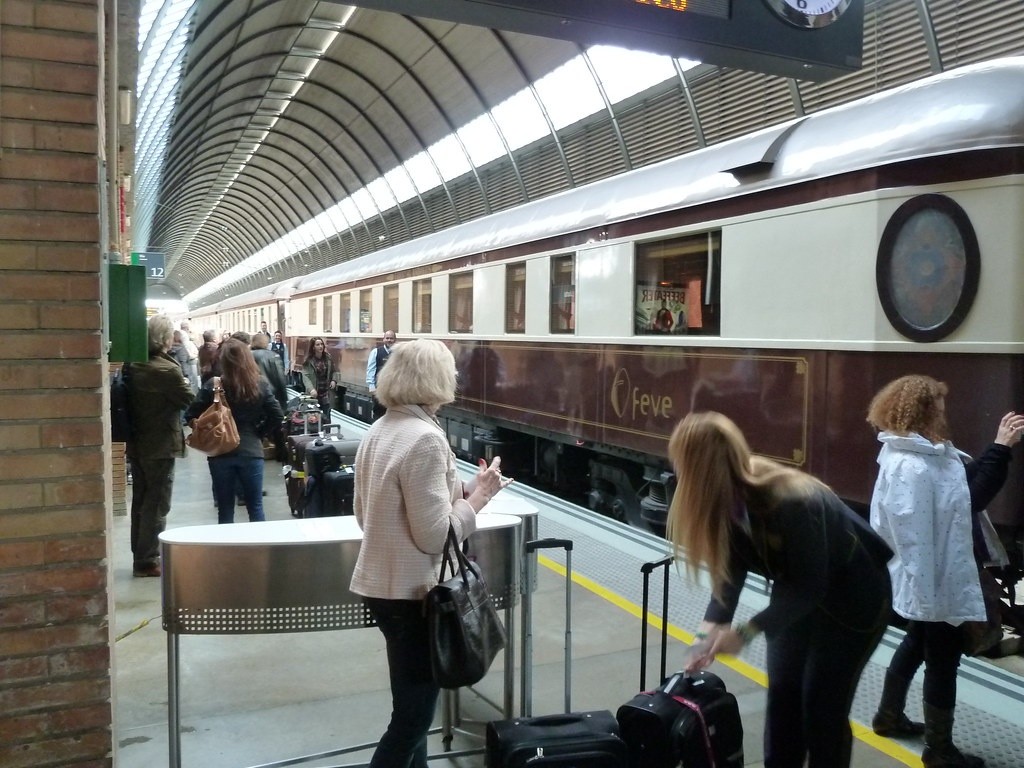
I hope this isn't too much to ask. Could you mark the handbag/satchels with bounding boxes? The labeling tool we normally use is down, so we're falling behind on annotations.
[423,521,508,690]
[184,376,240,457]
[961,549,1004,657]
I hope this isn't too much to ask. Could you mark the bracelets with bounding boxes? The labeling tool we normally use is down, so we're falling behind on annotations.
[694,629,707,640]
[735,622,755,642]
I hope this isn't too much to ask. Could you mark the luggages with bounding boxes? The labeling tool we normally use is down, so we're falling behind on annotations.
[323,463,356,518]
[616,556,744,768]
[287,392,331,434]
[303,424,361,518]
[283,410,332,518]
[485,538,630,768]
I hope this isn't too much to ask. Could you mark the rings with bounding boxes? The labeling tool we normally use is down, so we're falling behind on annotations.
[496,468,501,473]
[1010,426,1015,429]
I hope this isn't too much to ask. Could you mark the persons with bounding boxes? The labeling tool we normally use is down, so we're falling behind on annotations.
[349,340,514,768]
[366,330,396,422]
[167,321,289,524]
[866,376,1024,768]
[665,411,895,768]
[302,336,338,435]
[654,299,673,333]
[122,314,196,577]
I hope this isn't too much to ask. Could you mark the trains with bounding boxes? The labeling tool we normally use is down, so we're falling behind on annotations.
[176,55,1024,665]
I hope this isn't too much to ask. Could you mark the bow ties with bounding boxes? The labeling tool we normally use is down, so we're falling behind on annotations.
[276,342,282,345]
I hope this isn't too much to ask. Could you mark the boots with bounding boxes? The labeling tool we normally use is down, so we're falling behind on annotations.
[872,667,926,739]
[922,699,984,768]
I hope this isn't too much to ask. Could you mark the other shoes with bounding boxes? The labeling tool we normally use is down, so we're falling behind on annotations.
[262,491,267,496]
[213,500,218,507]
[238,500,246,506]
[153,560,160,568]
[133,567,161,577]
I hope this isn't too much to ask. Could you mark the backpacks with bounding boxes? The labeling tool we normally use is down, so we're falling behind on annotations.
[111,362,133,442]
